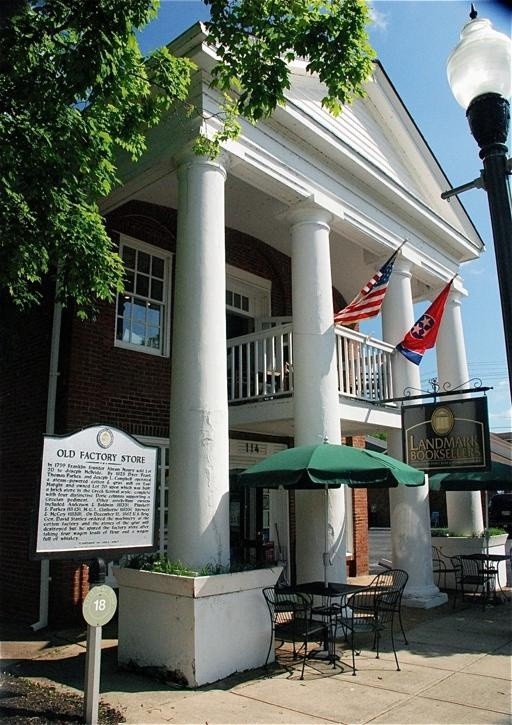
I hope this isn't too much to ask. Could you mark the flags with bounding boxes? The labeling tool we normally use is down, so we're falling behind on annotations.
[333,251,399,325]
[396,281,454,366]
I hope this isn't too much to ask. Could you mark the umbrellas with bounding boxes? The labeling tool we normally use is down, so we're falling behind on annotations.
[236,435,425,650]
[429,462,512,596]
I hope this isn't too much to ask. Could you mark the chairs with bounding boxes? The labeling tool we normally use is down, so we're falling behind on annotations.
[258,569,408,680]
[432,546,512,614]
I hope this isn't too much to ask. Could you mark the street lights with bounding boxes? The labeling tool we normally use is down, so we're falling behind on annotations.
[441,4,512,392]
